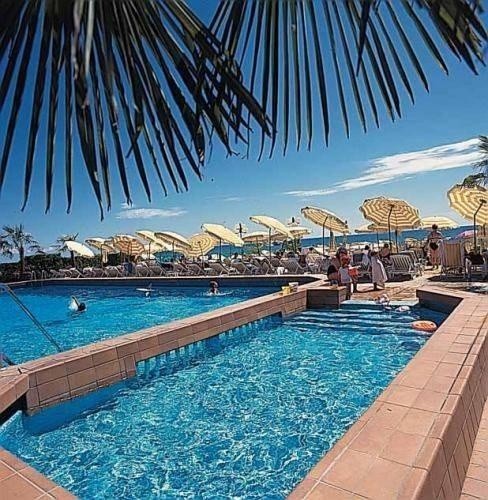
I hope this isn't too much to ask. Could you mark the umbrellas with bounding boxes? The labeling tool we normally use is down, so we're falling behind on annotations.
[446,182,488,227]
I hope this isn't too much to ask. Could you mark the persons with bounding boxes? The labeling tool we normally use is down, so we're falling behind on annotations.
[327,241,391,300]
[208,280,218,294]
[45,296,85,327]
[172,263,186,274]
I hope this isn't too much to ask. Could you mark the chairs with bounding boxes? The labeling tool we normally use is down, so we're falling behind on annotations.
[351,240,486,285]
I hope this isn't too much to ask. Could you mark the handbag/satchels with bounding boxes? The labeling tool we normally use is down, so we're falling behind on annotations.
[429,242,439,251]
